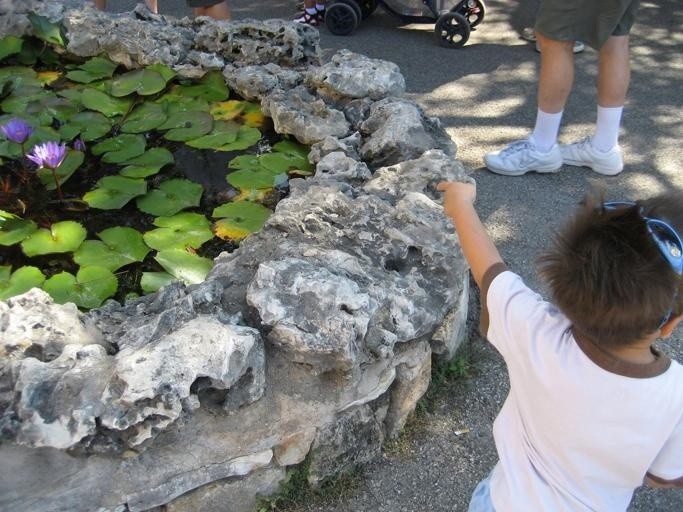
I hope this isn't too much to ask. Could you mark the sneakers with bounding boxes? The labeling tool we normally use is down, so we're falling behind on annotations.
[484,134,624,176]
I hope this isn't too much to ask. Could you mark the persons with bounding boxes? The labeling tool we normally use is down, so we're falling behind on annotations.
[94,0,327,27]
[484,0,635,176]
[435,180,683,512]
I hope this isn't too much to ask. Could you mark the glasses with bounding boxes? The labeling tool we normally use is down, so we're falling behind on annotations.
[602,201,683,329]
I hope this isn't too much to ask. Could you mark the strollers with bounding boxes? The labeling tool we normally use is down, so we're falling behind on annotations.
[324,0,486,49]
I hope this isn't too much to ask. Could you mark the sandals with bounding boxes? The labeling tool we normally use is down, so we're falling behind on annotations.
[293,7,326,27]
